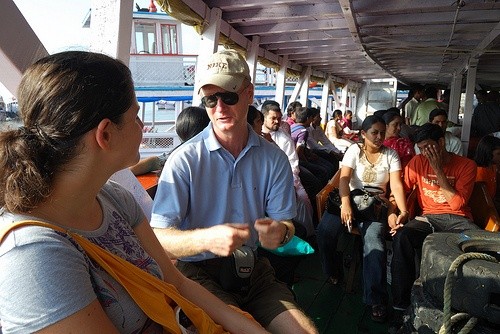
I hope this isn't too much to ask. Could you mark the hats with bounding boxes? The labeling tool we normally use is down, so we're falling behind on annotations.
[198,49,250,95]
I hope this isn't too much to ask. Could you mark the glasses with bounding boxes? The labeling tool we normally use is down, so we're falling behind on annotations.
[200,91,239,108]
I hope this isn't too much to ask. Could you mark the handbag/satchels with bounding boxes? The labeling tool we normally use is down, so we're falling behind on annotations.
[326,185,389,223]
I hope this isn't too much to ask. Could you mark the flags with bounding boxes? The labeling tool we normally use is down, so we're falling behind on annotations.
[82,10,92,27]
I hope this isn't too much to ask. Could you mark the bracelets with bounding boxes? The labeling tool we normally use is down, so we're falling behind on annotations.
[281,221,295,237]
[402,210,408,213]
[280,222,290,244]
[429,153,432,157]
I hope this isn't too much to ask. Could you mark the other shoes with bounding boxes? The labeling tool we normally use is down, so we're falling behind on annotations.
[389,307,406,327]
[369,304,386,321]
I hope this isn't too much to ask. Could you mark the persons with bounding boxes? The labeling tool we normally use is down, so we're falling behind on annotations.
[130,81,500,238]
[318,116,408,321]
[388,126,477,311]
[150,49,319,334]
[0,51,273,334]
